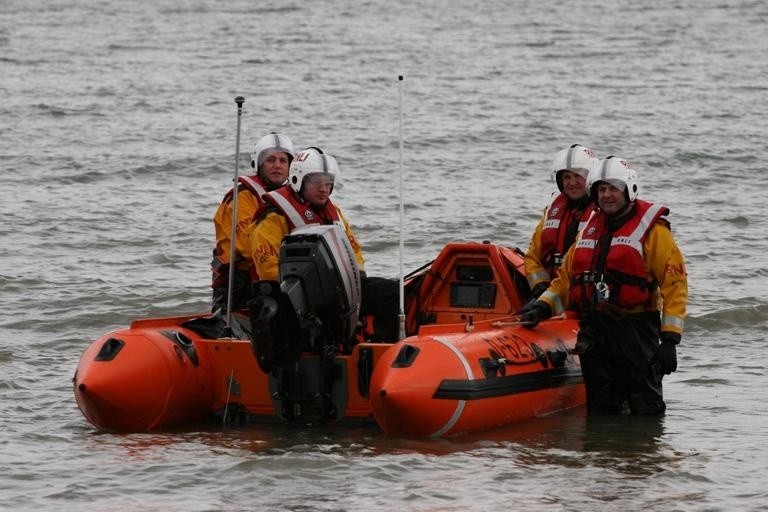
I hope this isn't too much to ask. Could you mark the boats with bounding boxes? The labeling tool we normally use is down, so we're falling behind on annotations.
[71,77,585,438]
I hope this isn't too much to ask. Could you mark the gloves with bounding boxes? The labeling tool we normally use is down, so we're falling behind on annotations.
[519,306,545,328]
[647,342,677,375]
[532,281,549,299]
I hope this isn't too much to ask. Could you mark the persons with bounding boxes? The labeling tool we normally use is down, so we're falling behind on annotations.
[521,142,603,297]
[208,132,298,316]
[249,145,368,334]
[517,153,690,419]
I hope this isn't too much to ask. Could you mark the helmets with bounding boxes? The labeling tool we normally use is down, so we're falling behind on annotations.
[249,133,294,176]
[552,144,598,193]
[583,155,639,202]
[286,146,340,193]
